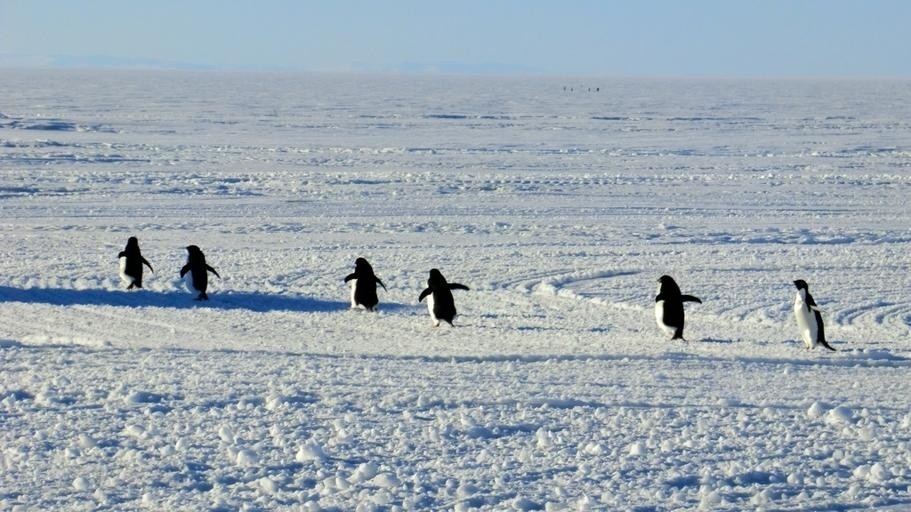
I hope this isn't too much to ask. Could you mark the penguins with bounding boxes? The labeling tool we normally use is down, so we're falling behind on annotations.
[654,275,702,342]
[343,257,387,314]
[179,245,220,301]
[118,237,153,290]
[418,269,470,327]
[793,279,837,352]
